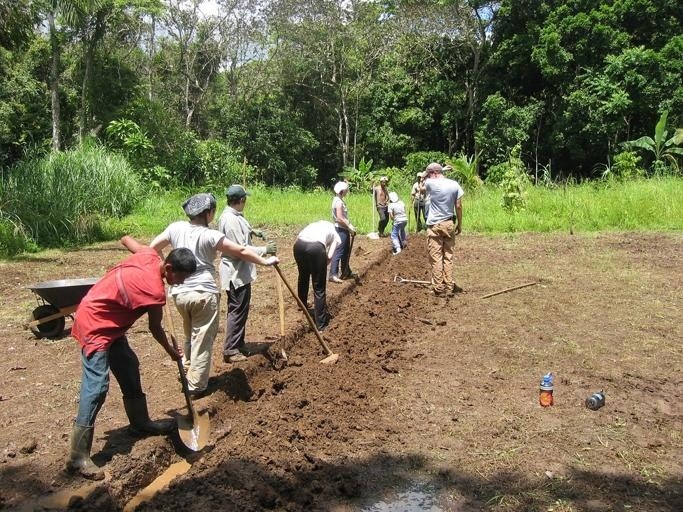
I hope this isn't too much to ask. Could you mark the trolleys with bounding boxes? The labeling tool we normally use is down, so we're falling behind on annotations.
[28,273,147,342]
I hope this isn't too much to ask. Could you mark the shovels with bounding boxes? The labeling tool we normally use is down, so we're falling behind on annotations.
[163,293,210,452]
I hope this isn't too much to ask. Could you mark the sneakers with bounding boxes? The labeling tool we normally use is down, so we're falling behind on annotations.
[329,277,342,282]
[342,274,358,279]
[224,352,248,363]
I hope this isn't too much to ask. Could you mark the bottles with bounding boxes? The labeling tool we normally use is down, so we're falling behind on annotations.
[584,389,605,410]
[538,373,553,407]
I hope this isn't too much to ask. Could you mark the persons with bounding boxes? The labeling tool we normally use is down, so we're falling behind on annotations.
[417,171,429,230]
[387,191,409,255]
[146,190,281,400]
[63,233,198,481]
[328,180,358,284]
[216,184,278,363]
[423,162,465,296]
[371,176,391,238]
[412,171,427,232]
[292,220,343,331]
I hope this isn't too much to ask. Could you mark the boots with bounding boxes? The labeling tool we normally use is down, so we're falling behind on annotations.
[123,395,175,434]
[66,422,105,481]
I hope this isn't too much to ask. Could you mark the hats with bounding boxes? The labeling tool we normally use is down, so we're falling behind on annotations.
[226,184,251,201]
